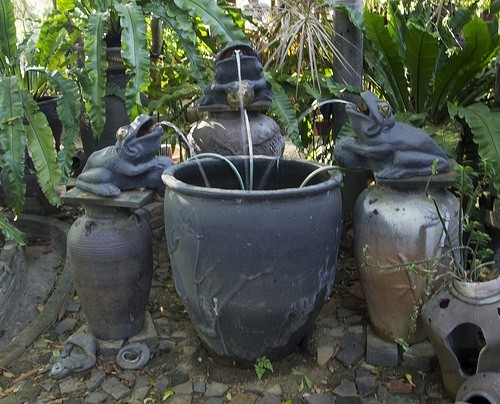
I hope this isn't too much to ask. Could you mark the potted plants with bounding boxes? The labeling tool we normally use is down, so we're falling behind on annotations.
[0,0,83,222]
[359,155,500,404]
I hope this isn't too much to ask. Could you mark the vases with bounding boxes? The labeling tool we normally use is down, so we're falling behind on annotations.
[456,371,500,404]
[189,111,284,157]
[66,203,151,341]
[356,182,465,345]
